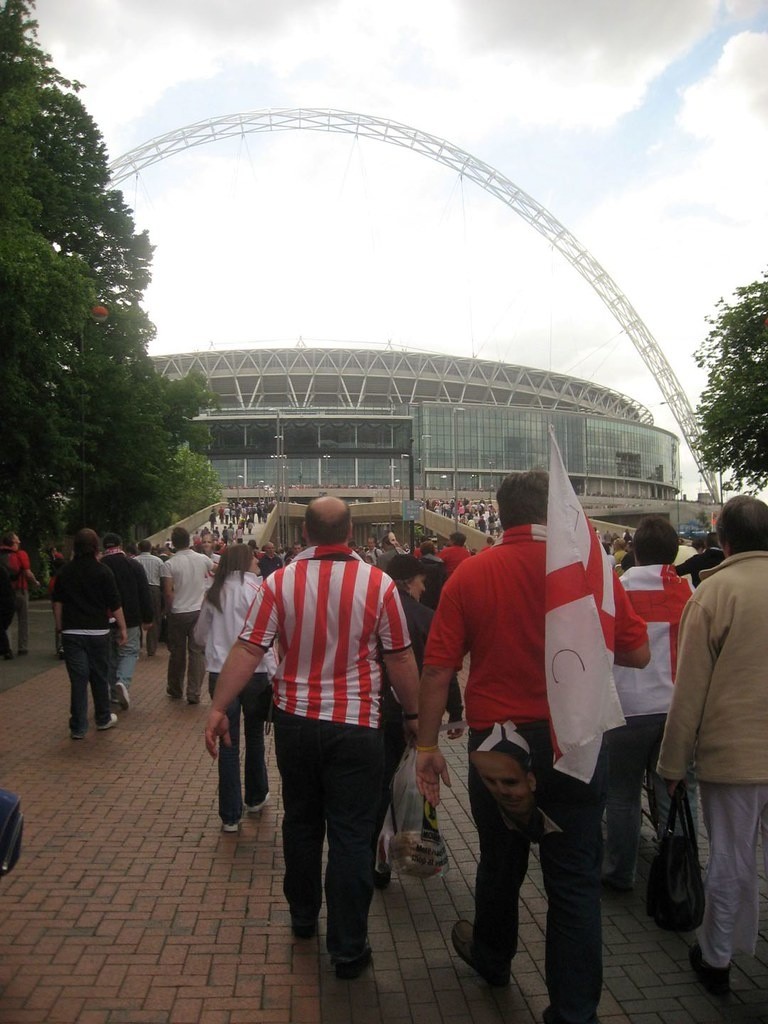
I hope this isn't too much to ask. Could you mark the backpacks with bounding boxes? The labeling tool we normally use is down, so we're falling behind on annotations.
[0,551,22,581]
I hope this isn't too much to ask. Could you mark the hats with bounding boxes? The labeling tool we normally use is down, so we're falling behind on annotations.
[104,532,122,548]
[386,554,426,579]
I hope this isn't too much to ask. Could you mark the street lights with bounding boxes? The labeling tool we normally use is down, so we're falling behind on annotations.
[401,453,409,546]
[455,408,465,531]
[323,453,330,486]
[274,435,283,488]
[236,475,244,503]
[420,434,433,536]
[258,480,265,505]
[441,475,448,504]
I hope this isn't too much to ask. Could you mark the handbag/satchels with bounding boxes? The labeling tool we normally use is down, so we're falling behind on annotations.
[374,735,449,876]
[0,790,25,877]
[646,780,705,931]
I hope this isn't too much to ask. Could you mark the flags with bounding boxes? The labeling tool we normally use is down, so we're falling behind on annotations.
[545,440,627,785]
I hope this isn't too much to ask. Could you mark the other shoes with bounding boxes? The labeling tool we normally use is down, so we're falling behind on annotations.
[68,729,83,739]
[97,713,117,730]
[167,685,183,698]
[111,682,129,710]
[603,878,633,892]
[688,944,730,995]
[187,695,200,705]
[292,924,315,942]
[372,866,391,884]
[245,790,269,813]
[18,647,28,654]
[223,815,243,831]
[335,948,371,979]
[5,649,13,659]
[57,647,65,659]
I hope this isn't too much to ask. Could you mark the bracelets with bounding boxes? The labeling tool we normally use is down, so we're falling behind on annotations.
[417,745,438,751]
[406,713,418,721]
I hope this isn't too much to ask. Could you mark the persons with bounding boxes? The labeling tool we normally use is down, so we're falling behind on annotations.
[0,531,41,661]
[194,545,281,833]
[374,555,467,888]
[420,494,505,537]
[208,498,276,546]
[50,525,222,741]
[471,746,557,840]
[416,469,651,1024]
[349,531,495,611]
[247,539,309,580]
[205,497,422,979]
[594,496,768,998]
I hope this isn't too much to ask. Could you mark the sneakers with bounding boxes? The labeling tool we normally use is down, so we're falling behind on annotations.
[452,918,502,983]
